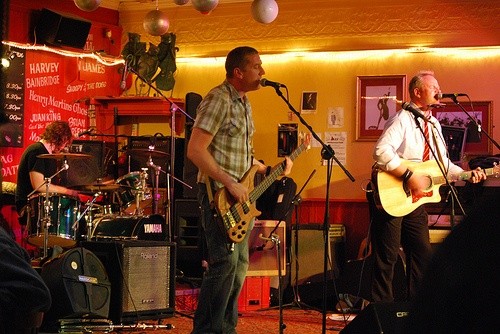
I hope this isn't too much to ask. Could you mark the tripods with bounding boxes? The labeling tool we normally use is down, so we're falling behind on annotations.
[257,168,324,312]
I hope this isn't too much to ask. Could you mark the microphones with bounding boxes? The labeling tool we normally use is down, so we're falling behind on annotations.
[121,60,127,89]
[438,93,466,98]
[251,245,272,250]
[260,79,286,88]
[402,102,429,122]
[74,128,93,137]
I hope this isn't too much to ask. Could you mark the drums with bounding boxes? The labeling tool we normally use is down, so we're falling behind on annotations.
[90,213,169,241]
[25,192,81,248]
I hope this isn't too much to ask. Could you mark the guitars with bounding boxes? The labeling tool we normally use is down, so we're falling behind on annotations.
[213,133,311,243]
[370,159,500,217]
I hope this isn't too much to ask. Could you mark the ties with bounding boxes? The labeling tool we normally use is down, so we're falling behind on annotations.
[423,120,430,162]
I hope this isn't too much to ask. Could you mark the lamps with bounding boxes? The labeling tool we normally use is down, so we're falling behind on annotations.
[70,0,278,37]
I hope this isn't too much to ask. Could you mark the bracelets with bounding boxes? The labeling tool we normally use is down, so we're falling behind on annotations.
[400,169,413,182]
[265,166,270,176]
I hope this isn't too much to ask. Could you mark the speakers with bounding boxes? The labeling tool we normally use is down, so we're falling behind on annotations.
[245,220,286,276]
[175,199,208,281]
[289,224,332,287]
[80,238,176,322]
[339,302,443,334]
[34,8,92,50]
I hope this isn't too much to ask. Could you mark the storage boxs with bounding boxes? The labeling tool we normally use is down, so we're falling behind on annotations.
[236,277,271,313]
[173,281,201,313]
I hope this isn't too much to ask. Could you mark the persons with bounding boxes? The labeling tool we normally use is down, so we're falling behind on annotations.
[0,214,51,334]
[15,122,92,233]
[368,72,486,312]
[188,45,294,334]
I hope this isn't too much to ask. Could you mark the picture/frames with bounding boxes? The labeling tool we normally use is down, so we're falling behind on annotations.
[431,100,493,158]
[356,74,407,142]
